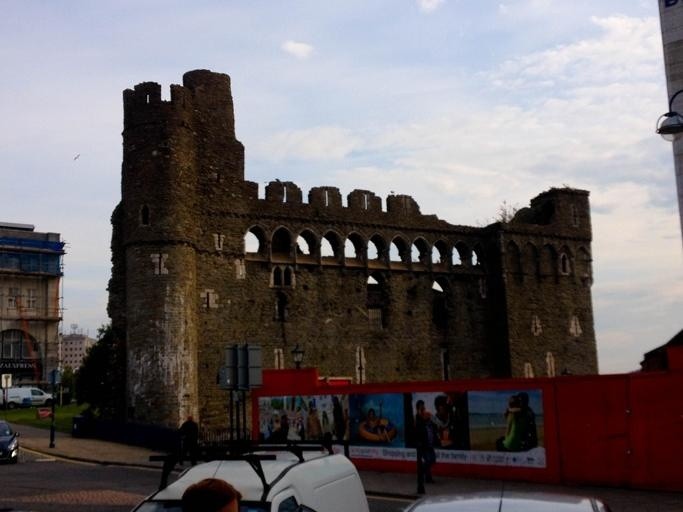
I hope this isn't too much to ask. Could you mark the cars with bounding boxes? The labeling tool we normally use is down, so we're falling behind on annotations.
[404,489,604,512]
[0,421,20,465]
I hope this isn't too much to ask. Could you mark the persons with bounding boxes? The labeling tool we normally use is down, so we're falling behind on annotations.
[259,396,350,443]
[181,479,242,512]
[415,400,438,483]
[432,392,468,450]
[495,393,538,451]
[366,408,393,443]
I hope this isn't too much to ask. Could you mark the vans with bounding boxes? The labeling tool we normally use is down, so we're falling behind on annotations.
[5,388,53,409]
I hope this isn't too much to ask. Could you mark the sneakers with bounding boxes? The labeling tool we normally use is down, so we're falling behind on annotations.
[417,479,435,494]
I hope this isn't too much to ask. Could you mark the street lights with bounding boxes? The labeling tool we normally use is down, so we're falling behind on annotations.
[49,369,56,447]
[291,343,304,368]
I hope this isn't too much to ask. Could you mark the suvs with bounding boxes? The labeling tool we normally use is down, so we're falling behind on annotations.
[131,440,369,512]
